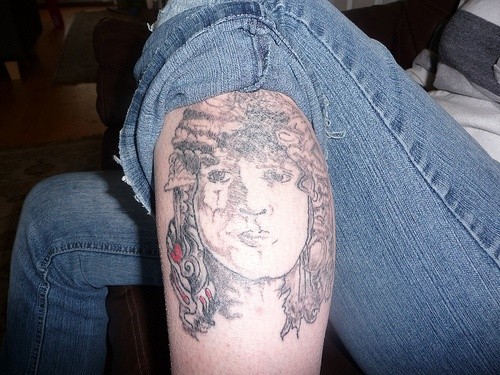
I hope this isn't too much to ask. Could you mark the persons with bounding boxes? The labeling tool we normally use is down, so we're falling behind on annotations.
[0,0,500,374]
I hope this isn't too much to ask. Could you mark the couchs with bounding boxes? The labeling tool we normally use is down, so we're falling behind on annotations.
[94,1,460,375]
